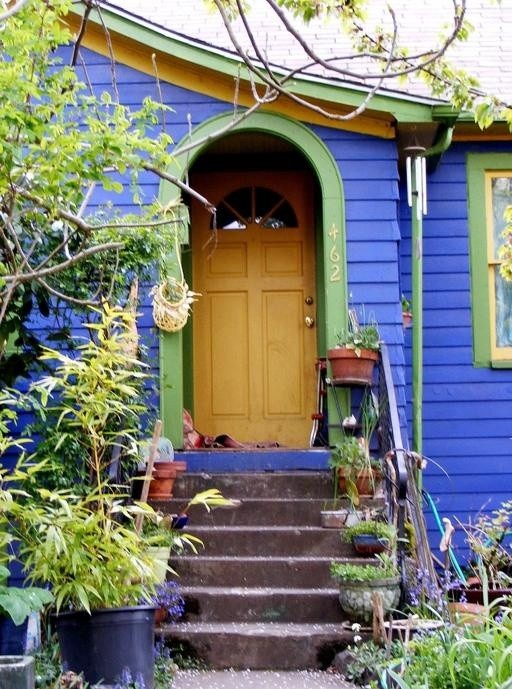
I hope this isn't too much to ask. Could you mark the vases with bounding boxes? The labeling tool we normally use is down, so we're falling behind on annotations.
[147,471,178,501]
[145,461,187,471]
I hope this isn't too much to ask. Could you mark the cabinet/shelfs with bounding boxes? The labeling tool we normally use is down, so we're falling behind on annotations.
[326,377,373,438]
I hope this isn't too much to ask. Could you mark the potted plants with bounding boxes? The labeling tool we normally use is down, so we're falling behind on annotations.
[327,327,379,383]
[0,294,242,688]
[445,499,511,619]
[338,520,398,557]
[328,551,403,625]
[402,293,412,326]
[325,436,381,508]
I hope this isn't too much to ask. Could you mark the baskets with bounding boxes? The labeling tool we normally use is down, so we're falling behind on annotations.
[152,281,189,333]
